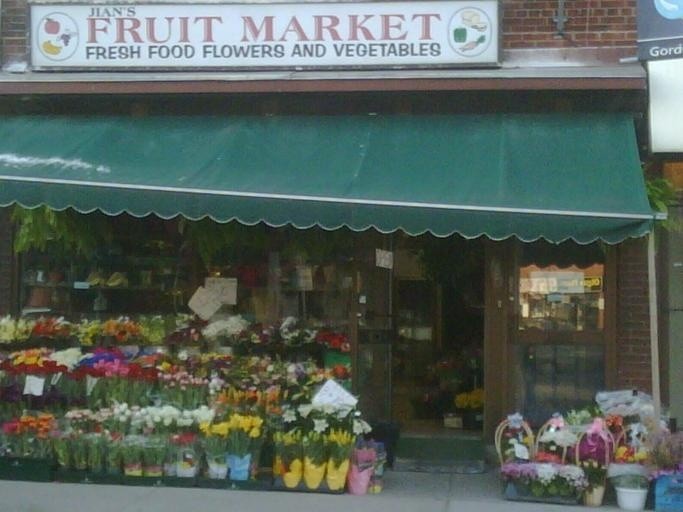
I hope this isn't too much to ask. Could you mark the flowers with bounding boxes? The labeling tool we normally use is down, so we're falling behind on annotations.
[495,406,683,498]
[410,354,485,412]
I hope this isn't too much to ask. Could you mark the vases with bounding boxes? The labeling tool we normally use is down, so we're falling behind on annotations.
[443,413,474,432]
[159,273,178,292]
[582,485,649,510]
[241,266,259,287]
[26,270,64,307]
[293,266,314,290]
